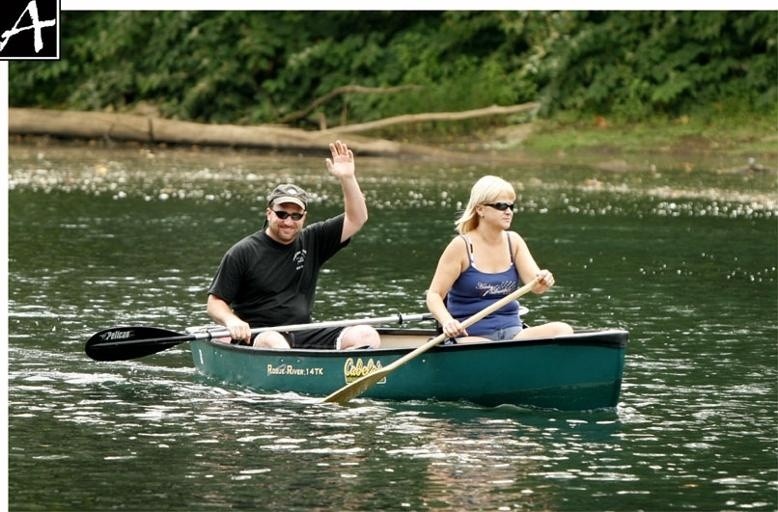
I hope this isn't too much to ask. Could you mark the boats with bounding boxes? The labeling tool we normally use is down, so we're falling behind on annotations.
[183,325,631,410]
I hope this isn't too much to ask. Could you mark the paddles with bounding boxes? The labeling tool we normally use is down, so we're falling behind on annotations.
[85,314,438,361]
[321,276,540,402]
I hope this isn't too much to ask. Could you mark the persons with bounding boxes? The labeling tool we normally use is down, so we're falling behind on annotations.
[206,139,381,348]
[425,173,574,344]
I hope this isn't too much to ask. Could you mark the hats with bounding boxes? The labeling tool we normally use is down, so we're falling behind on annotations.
[268,184,307,210]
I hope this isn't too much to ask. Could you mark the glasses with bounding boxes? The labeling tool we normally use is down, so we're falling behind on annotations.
[484,203,513,210]
[273,210,303,220]
